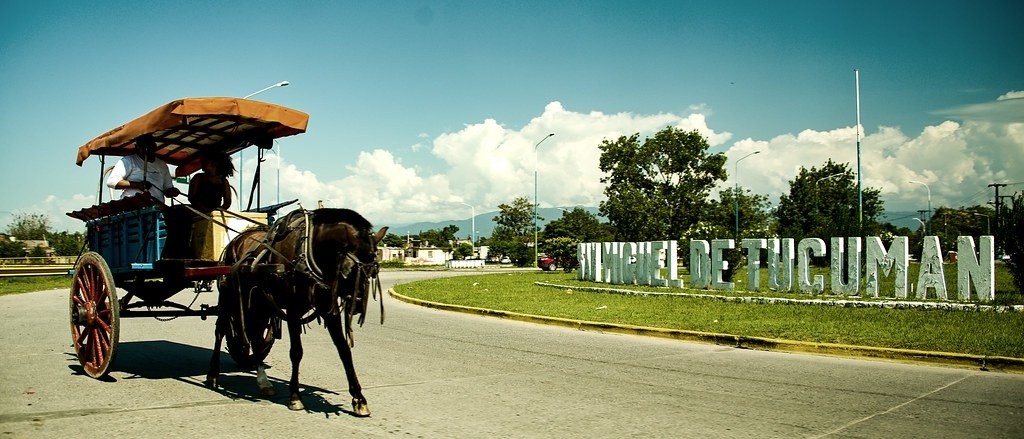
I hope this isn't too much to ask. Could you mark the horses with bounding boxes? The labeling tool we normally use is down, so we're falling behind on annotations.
[209,208,389,419]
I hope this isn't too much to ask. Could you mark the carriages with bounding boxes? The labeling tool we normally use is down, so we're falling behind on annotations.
[69,97,390,418]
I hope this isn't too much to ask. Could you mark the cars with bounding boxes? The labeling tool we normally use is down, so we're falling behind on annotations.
[538,255,559,271]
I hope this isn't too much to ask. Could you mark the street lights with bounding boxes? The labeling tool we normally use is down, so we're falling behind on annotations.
[816,172,844,210]
[459,202,475,256]
[533,133,555,268]
[909,180,931,236]
[239,80,292,215]
[734,150,761,238]
[974,212,991,235]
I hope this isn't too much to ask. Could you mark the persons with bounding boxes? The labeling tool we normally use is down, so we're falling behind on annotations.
[106,135,180,204]
[188,152,234,214]
